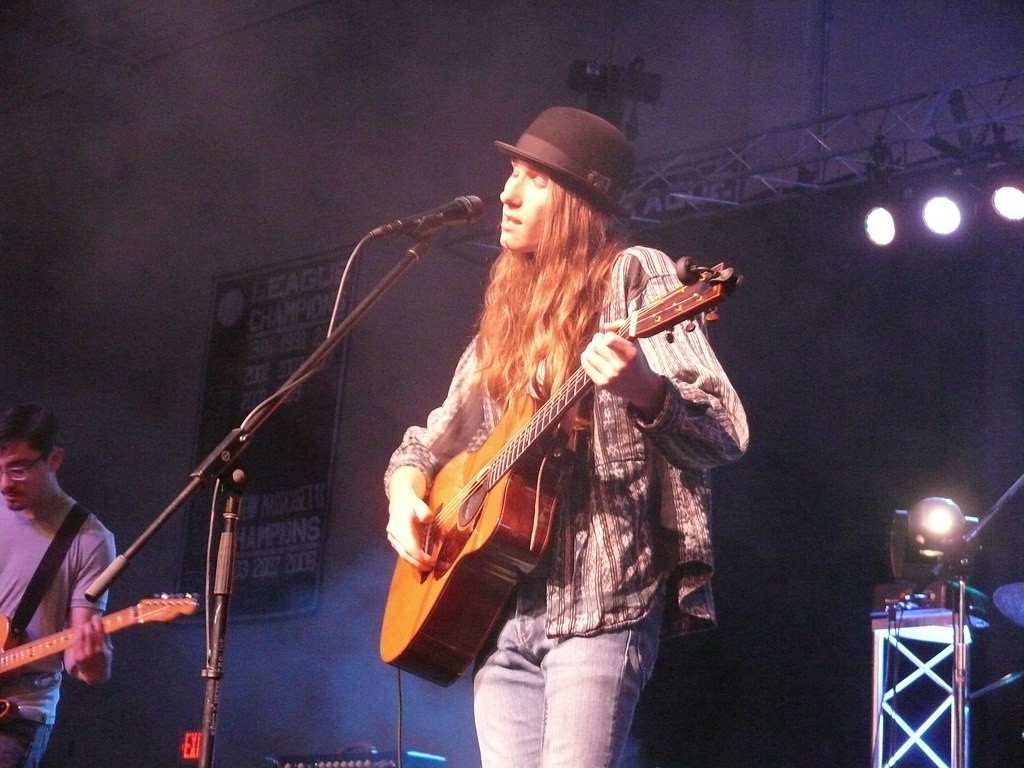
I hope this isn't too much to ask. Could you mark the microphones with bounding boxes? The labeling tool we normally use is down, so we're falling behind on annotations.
[368,195,484,239]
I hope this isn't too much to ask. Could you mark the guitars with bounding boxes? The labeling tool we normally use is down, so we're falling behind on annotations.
[377,255,746,689]
[0,591,200,727]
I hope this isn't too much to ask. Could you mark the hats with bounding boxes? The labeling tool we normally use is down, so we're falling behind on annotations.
[493,105,634,220]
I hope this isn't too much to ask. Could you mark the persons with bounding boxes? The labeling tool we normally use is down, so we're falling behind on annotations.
[376,109,747,768]
[0,402,108,768]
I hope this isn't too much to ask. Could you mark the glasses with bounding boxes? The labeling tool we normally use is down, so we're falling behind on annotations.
[0,455,42,481]
